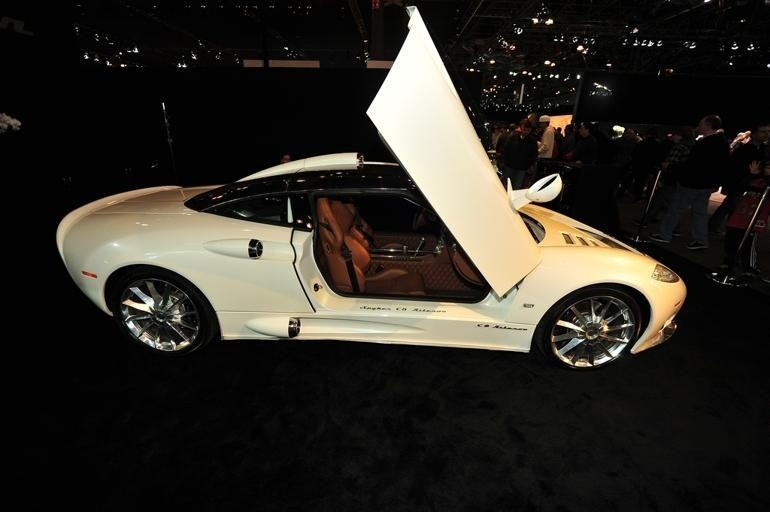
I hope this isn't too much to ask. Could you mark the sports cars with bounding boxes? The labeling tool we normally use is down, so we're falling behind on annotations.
[50,6,687,377]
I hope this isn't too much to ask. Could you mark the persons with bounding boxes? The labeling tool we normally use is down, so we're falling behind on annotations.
[722,123,770,287]
[480,111,752,252]
[278,151,291,164]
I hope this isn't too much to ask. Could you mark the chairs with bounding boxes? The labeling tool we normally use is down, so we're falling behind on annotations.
[317,198,426,297]
